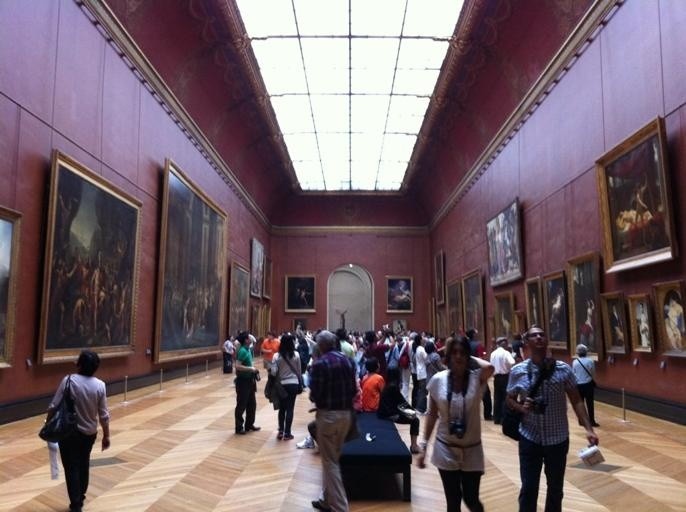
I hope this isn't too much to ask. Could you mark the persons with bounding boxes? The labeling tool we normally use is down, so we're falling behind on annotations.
[572,344,599,427]
[632,172,650,252]
[390,279,411,309]
[357,328,447,454]
[580,299,594,346]
[466,329,528,425]
[222,324,358,449]
[506,326,600,511]
[417,334,495,511]
[165,280,216,340]
[663,291,684,348]
[610,305,625,347]
[52,246,129,349]
[46,350,111,512]
[308,329,358,511]
[639,302,651,347]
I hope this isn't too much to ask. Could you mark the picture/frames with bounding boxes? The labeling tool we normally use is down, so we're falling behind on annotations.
[567,248,602,363]
[462,265,485,342]
[650,279,686,352]
[384,274,413,315]
[0,205,24,369]
[595,113,679,275]
[37,147,144,368]
[283,274,317,314]
[262,254,273,300]
[492,290,515,339]
[433,249,445,306]
[444,276,463,336]
[251,236,265,298]
[523,275,545,330]
[626,293,654,354]
[542,269,568,350]
[599,289,629,355]
[228,257,251,337]
[484,196,524,287]
[153,157,229,367]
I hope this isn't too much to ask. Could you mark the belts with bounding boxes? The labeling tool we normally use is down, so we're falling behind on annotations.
[436,437,481,449]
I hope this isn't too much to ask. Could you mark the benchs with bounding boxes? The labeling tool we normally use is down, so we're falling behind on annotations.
[340,411,413,502]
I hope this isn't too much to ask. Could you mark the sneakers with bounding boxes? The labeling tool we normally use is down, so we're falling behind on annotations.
[277,432,283,439]
[296,439,315,449]
[312,500,326,510]
[284,433,293,440]
[484,414,493,420]
[590,421,600,427]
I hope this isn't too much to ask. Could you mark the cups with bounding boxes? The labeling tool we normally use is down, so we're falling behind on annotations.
[579,444,605,467]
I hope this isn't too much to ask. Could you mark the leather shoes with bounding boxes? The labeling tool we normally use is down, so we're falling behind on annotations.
[245,426,260,431]
[235,430,246,435]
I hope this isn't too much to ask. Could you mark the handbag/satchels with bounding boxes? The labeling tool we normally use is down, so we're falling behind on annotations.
[298,377,304,394]
[398,406,416,419]
[500,401,520,440]
[344,416,359,442]
[38,386,78,443]
[400,353,410,369]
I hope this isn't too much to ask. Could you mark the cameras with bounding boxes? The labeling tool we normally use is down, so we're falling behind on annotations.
[530,396,548,414]
[448,418,466,438]
[253,369,260,381]
